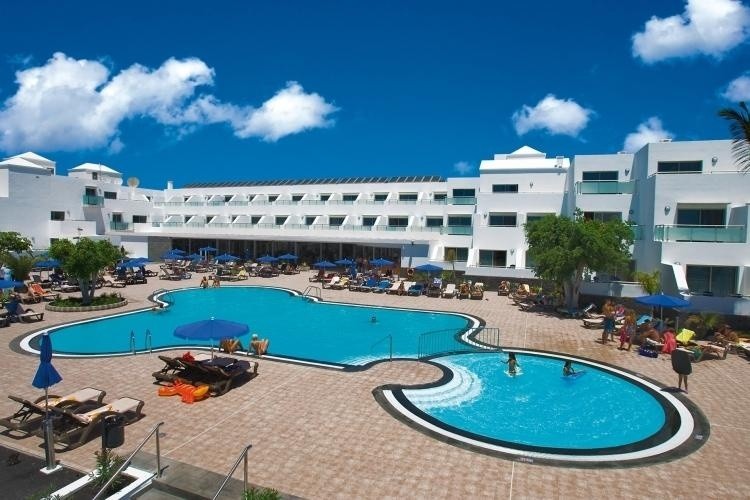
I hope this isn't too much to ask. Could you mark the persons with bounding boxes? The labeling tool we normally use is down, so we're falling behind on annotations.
[209,267,223,280]
[236,265,247,280]
[457,279,539,311]
[200,275,209,289]
[582,297,740,355]
[499,352,520,374]
[116,267,146,285]
[396,280,405,296]
[241,333,270,359]
[8,275,53,305]
[211,275,220,288]
[367,315,381,323]
[562,359,576,377]
[217,334,245,355]
[672,344,692,393]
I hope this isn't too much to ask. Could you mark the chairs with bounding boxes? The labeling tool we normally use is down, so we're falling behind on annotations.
[582,306,749,362]
[498,281,555,312]
[556,303,596,319]
[95,267,192,289]
[309,270,484,301]
[152,355,258,405]
[1,268,80,327]
[196,265,301,281]
[1,387,145,450]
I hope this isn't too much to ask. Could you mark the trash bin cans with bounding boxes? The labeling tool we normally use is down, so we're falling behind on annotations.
[105,416,124,448]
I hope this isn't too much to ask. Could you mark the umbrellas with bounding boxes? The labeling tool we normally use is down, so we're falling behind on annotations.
[32,258,62,282]
[0,279,24,303]
[172,316,249,362]
[311,256,395,282]
[113,245,299,276]
[634,289,692,333]
[31,330,63,418]
[413,264,443,281]
